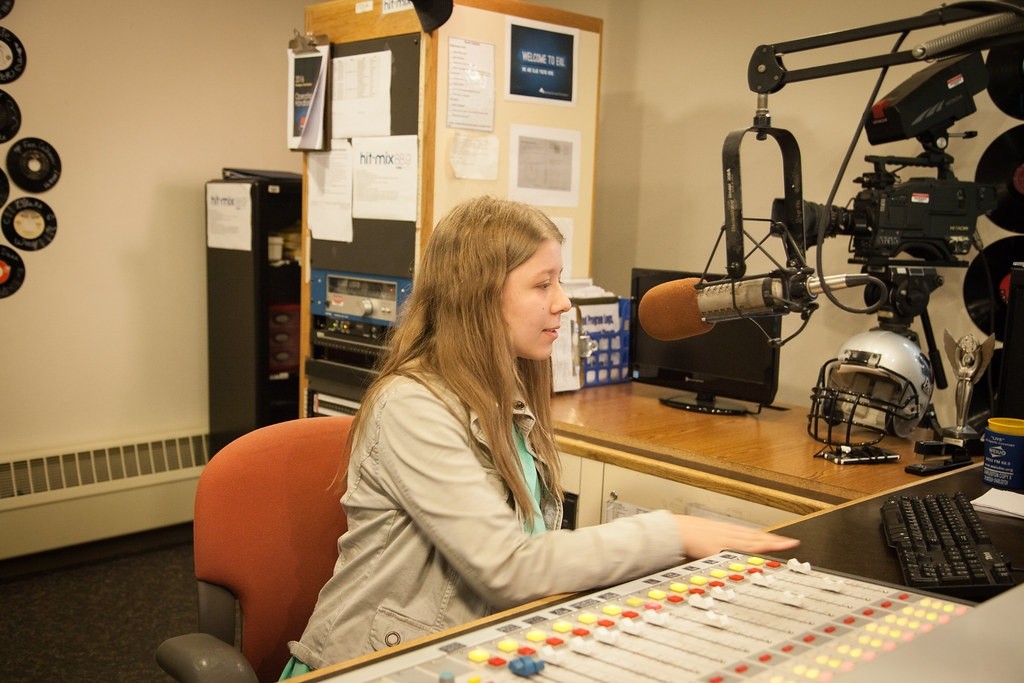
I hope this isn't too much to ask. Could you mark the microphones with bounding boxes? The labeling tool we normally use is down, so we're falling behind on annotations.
[638,271,870,341]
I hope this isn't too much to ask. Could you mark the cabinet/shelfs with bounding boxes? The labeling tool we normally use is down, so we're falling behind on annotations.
[297,0,605,417]
[543,384,980,533]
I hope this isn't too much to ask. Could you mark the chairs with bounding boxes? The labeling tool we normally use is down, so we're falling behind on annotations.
[155,417,360,683]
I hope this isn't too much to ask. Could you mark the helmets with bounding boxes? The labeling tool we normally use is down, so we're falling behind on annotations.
[808,330,933,447]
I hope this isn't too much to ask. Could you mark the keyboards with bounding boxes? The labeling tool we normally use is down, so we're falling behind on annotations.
[880,489,1012,594]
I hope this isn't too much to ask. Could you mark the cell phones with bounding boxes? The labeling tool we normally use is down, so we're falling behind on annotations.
[823,447,901,464]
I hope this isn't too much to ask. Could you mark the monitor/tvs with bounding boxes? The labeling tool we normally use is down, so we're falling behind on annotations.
[628,268,782,416]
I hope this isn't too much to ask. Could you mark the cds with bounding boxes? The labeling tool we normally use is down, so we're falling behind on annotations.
[0,0,62,298]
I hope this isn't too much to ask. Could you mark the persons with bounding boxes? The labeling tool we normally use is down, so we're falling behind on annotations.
[278,198,801,683]
